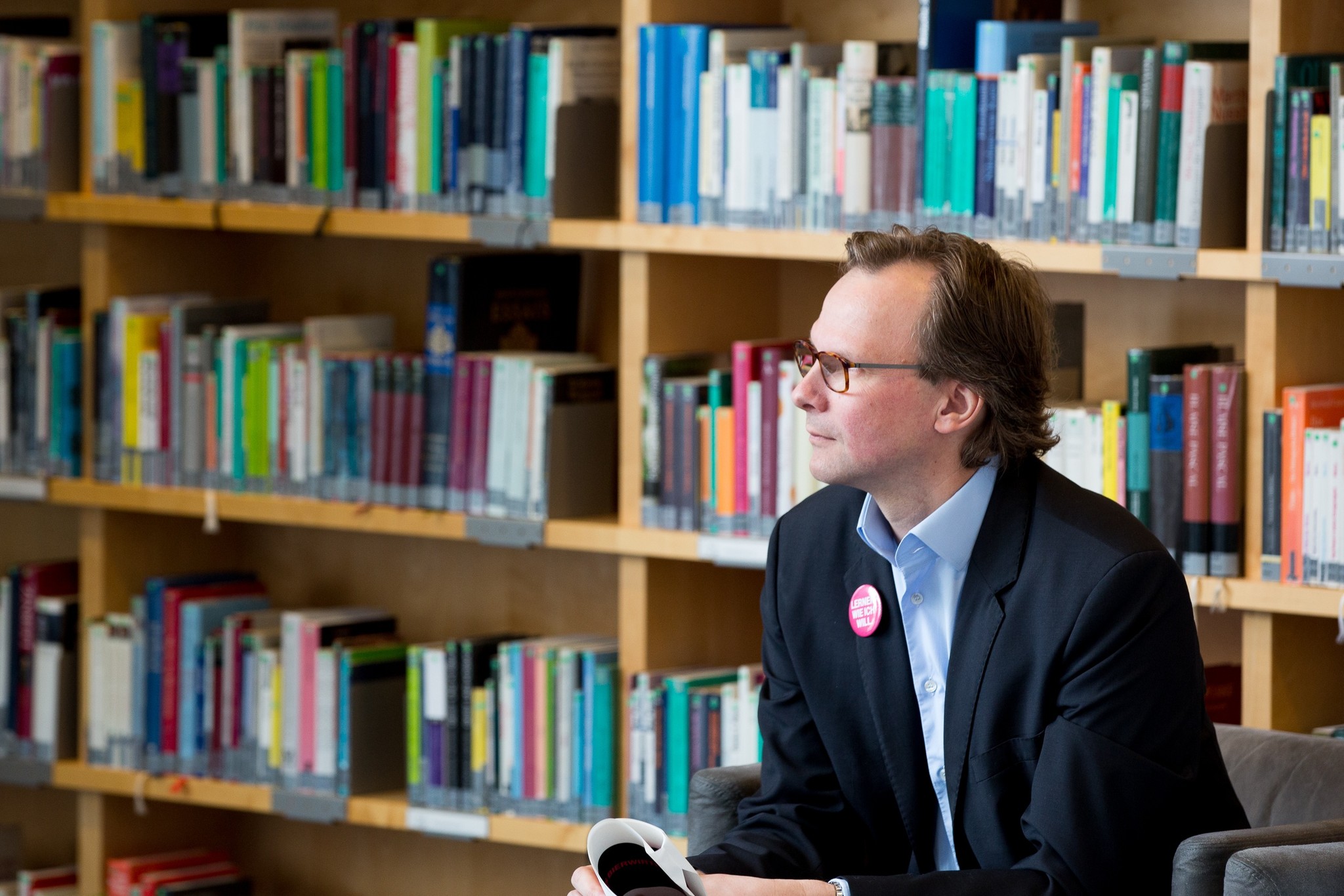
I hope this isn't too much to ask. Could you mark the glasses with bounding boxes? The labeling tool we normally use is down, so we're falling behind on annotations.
[794,340,937,394]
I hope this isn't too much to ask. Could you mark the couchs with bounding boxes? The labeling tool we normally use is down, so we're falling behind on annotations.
[687,722,1344,896]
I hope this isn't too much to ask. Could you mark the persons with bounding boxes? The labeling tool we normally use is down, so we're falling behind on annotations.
[566,224,1250,896]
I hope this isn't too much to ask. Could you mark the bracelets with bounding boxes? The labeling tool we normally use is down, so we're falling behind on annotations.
[829,881,844,896]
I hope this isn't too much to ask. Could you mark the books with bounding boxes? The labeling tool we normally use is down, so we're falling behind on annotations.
[1,0,1343,896]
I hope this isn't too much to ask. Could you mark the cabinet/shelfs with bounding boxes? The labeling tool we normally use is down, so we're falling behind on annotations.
[0,0,1344,896]
